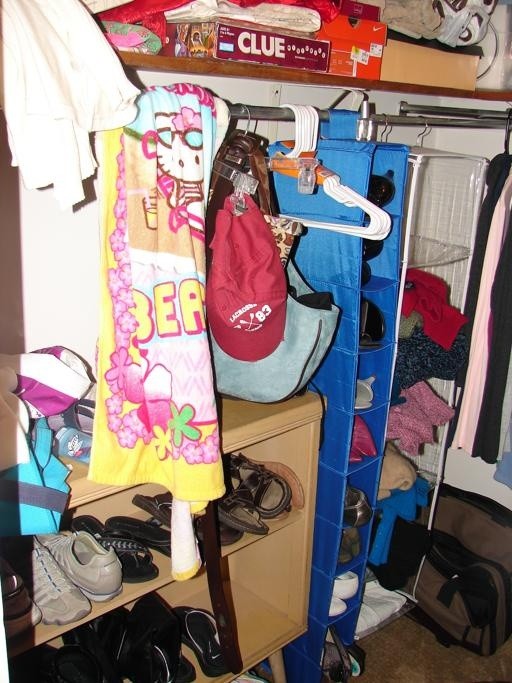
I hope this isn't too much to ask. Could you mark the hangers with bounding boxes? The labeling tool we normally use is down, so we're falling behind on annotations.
[267,103,392,243]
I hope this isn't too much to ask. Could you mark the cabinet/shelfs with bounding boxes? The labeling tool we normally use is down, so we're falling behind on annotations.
[354,147,489,639]
[264,142,407,683]
[1,390,325,683]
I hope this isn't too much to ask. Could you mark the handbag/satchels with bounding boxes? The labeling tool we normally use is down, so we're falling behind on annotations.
[209,255,340,404]
[403,527,511,656]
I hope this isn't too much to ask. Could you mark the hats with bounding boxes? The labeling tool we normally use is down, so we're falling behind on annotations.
[208,194,287,362]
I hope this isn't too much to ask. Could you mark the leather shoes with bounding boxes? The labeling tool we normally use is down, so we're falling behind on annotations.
[319,171,395,680]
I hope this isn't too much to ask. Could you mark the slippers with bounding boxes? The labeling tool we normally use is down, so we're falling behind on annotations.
[48,398,100,435]
